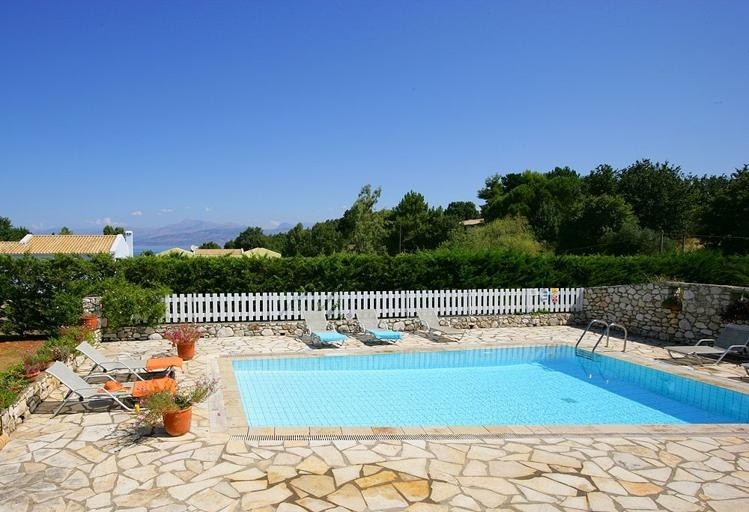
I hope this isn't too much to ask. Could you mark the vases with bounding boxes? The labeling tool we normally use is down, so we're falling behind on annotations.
[80,316,97,329]
[178,343,194,360]
[162,405,193,437]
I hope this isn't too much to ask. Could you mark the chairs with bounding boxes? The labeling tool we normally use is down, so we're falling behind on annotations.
[298,308,467,348]
[663,322,749,382]
[44,341,183,419]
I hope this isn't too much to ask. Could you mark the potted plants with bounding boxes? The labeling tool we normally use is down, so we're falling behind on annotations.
[9,363,41,384]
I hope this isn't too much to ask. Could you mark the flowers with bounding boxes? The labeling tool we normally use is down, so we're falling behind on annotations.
[119,374,224,443]
[159,324,206,348]
